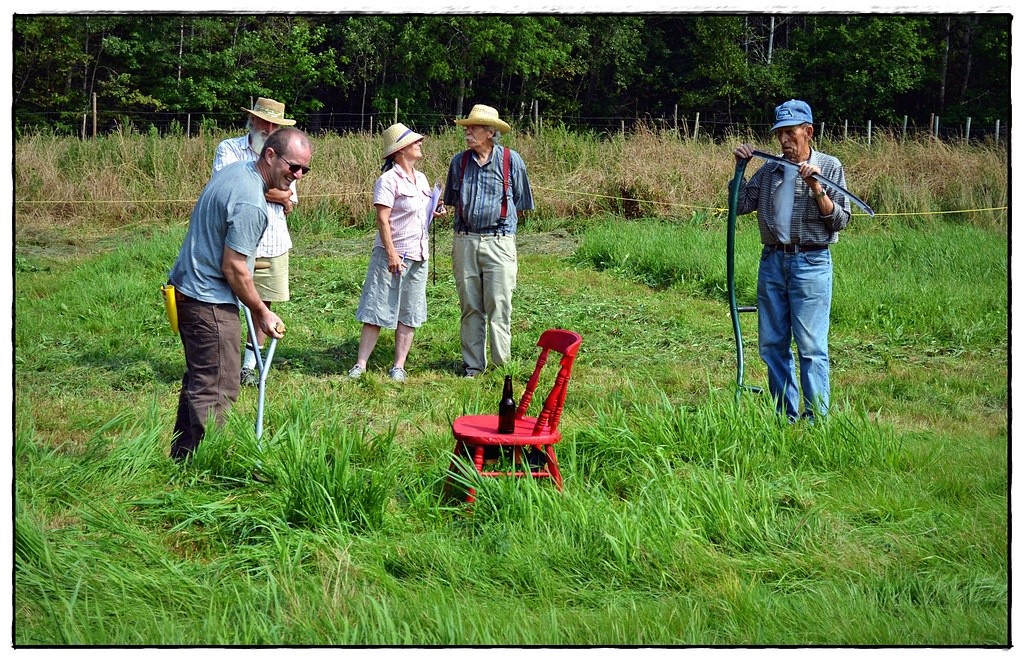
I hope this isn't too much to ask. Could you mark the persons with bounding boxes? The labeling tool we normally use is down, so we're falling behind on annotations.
[163,126,313,457]
[441,102,536,383]
[209,95,300,389]
[729,98,852,430]
[345,121,448,381]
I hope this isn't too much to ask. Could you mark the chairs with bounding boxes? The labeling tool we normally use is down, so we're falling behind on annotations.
[439,328,582,515]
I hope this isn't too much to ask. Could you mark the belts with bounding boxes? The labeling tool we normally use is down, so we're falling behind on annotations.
[768,241,828,253]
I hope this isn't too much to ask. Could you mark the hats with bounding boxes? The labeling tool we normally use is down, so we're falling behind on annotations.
[454,104,510,135]
[240,97,297,126]
[769,99,813,131]
[380,122,425,159]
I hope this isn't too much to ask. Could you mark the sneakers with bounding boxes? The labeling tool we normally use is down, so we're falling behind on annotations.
[390,367,406,381]
[348,364,366,379]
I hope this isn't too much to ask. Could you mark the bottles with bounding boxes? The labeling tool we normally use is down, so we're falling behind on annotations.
[497,375,518,434]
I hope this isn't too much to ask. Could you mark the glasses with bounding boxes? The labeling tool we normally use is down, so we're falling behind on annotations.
[272,149,311,175]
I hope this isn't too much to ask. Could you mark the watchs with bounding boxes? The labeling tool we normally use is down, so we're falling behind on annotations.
[812,187,827,202]
